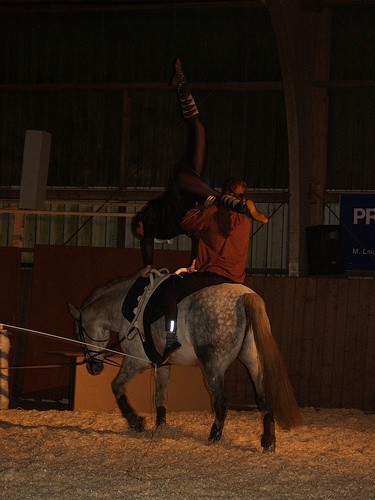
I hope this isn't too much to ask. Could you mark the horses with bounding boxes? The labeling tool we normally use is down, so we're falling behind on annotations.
[66,266,310,454]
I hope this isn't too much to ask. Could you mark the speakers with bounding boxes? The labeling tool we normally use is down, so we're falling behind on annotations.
[305,225,344,275]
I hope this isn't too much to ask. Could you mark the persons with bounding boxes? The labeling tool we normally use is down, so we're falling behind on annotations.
[131,54,268,277]
[159,180,251,359]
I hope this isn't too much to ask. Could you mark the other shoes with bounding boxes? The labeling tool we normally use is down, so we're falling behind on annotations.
[241,199,269,224]
[168,57,185,89]
[159,341,182,365]
